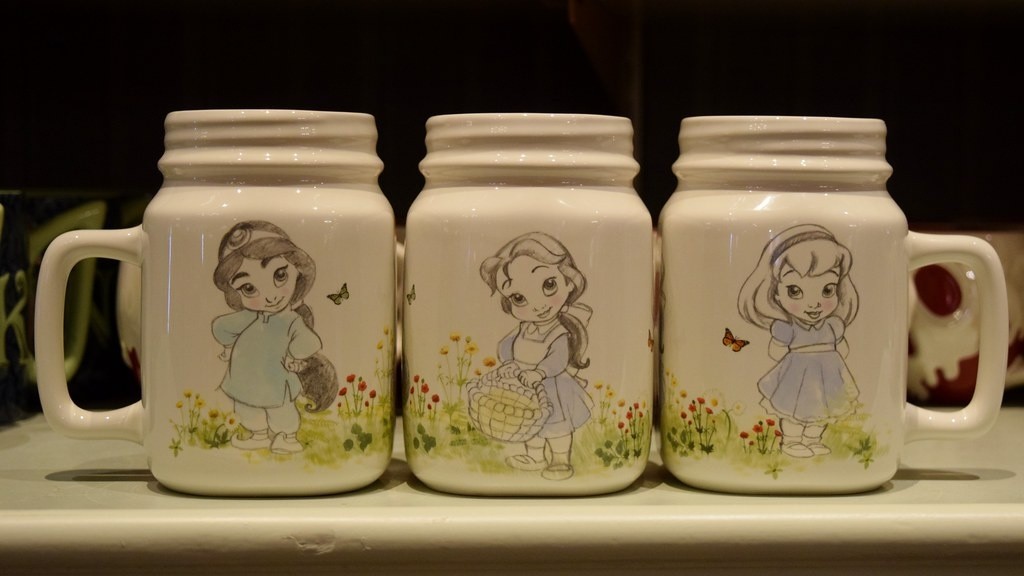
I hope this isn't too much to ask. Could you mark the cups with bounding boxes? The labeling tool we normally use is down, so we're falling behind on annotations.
[0,190,106,427]
[403,113,654,497]
[658,116,1009,495]
[35,110,395,496]
[909,233,1024,407]
